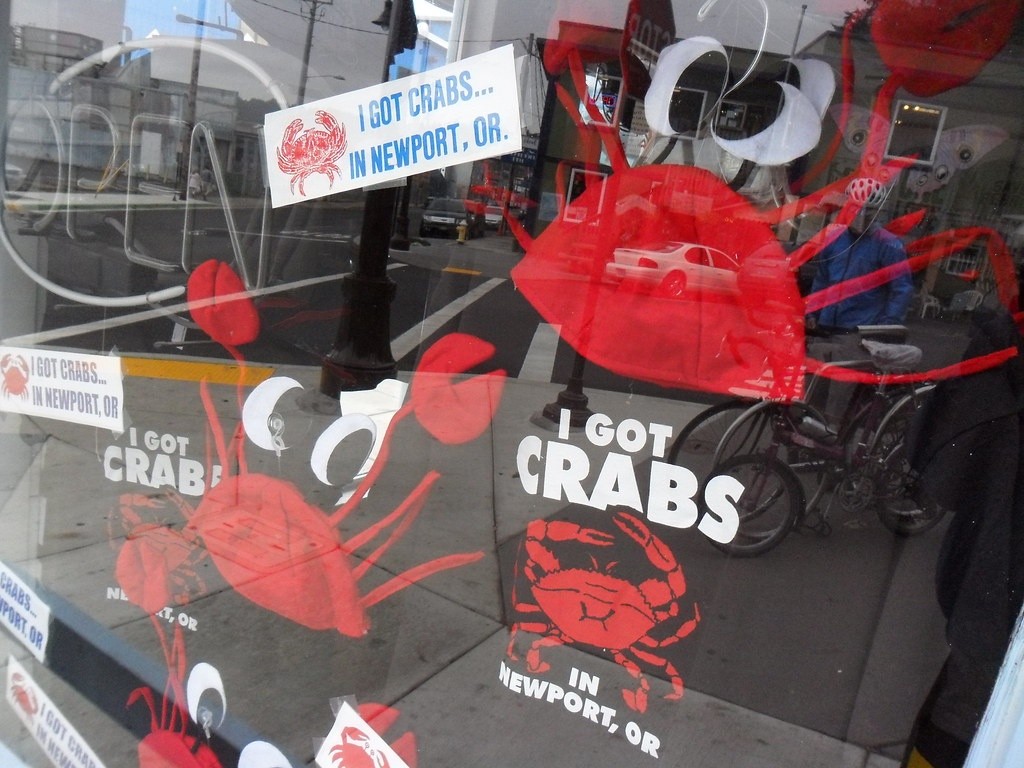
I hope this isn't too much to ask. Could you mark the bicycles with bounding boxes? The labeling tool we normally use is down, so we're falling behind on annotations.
[661,324,952,559]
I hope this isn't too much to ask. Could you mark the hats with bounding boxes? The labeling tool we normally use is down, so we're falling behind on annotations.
[845,177,887,208]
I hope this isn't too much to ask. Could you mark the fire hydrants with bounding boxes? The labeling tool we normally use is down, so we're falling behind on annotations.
[455,219,469,244]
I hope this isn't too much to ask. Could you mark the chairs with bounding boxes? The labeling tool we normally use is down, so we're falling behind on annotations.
[916,280,941,319]
[961,290,982,312]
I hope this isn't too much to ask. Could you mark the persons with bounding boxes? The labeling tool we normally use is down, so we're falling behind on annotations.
[189,165,215,201]
[787,178,914,491]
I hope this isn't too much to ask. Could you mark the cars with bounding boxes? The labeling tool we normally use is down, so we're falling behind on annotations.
[482,205,507,230]
[420,198,485,241]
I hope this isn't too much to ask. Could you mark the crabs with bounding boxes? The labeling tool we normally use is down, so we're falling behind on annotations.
[110,255,507,768]
[457,1,1024,407]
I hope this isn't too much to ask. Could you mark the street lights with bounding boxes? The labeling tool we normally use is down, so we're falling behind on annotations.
[172,14,245,175]
[321,0,420,400]
[297,74,344,109]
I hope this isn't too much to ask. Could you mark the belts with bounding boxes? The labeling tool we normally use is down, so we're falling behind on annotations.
[824,325,850,334]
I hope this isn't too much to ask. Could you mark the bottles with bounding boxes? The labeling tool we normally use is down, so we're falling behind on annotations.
[792,415,839,445]
